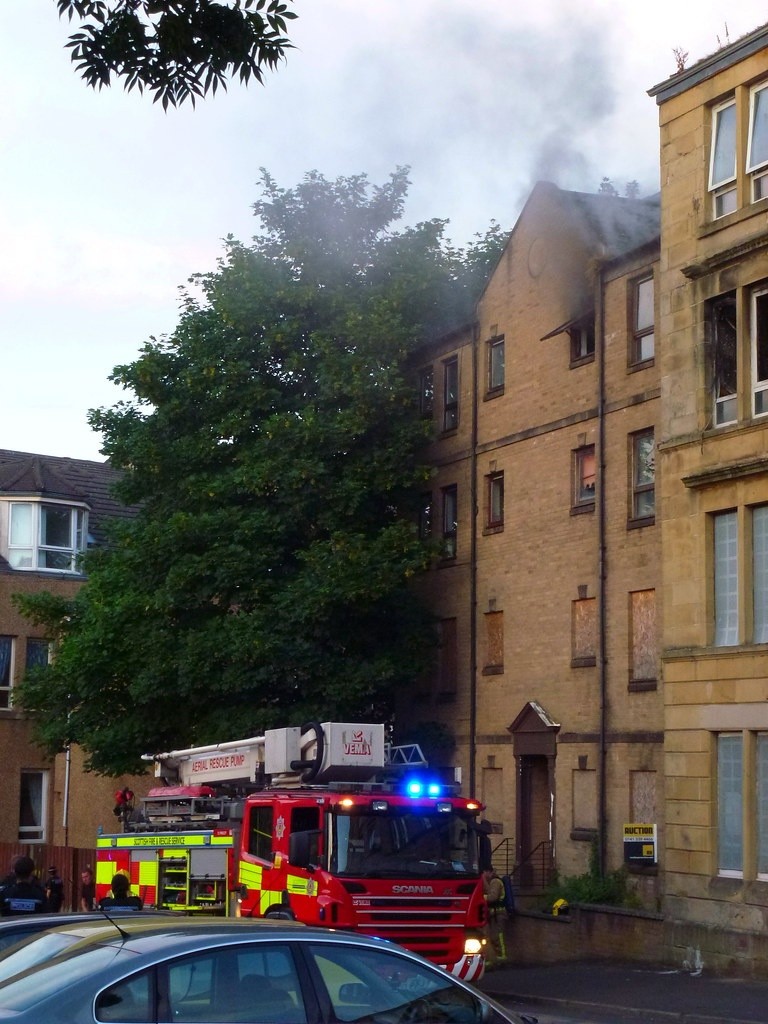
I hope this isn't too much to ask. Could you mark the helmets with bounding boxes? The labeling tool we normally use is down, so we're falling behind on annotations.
[115,868,131,884]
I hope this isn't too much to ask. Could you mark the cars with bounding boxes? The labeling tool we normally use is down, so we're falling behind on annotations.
[0,913,536,1023]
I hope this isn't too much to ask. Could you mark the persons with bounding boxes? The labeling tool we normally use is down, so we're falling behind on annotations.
[44,866,66,913]
[0,856,44,916]
[79,869,96,913]
[99,874,144,911]
[482,865,509,969]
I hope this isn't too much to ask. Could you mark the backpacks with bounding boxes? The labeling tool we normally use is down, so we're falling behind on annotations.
[492,875,515,920]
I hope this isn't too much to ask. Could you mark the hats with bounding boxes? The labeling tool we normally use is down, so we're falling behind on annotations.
[112,874,129,892]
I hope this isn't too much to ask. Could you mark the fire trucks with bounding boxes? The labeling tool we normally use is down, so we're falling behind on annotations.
[88,723,492,984]
